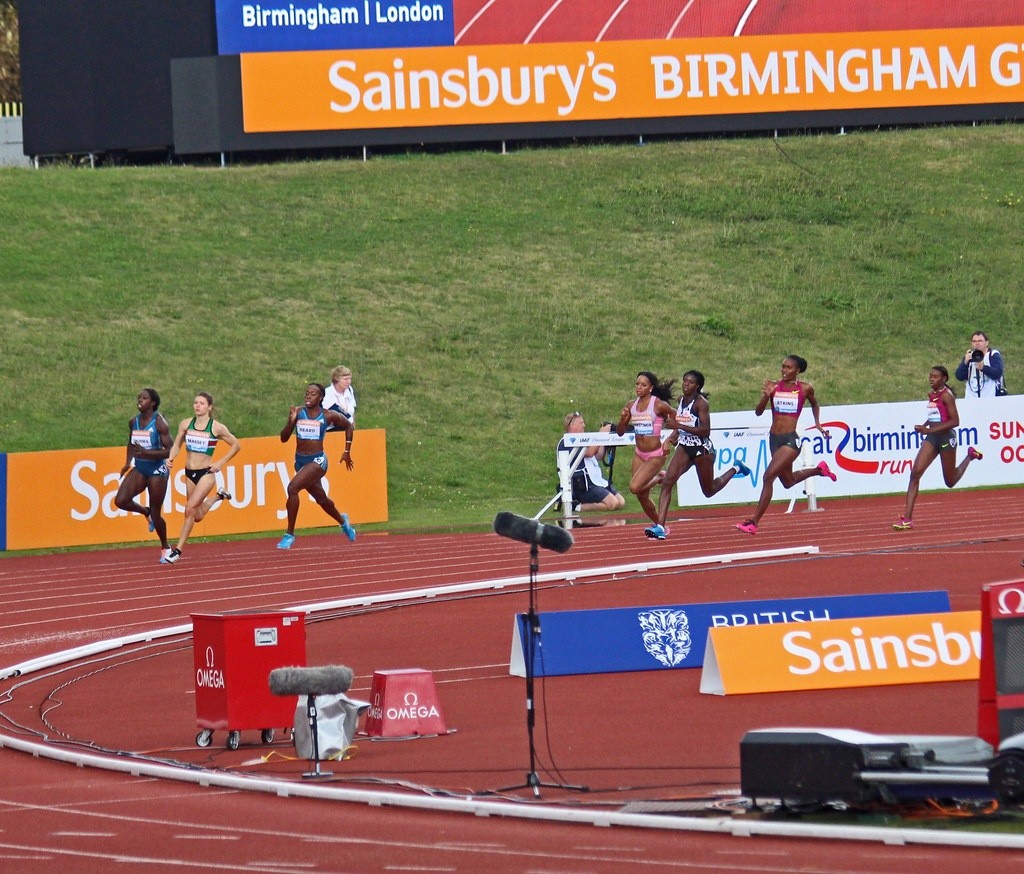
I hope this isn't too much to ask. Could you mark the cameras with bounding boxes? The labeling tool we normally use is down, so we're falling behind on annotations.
[968,350,984,362]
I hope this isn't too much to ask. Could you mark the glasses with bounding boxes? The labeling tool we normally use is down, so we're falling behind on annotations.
[567,411,580,428]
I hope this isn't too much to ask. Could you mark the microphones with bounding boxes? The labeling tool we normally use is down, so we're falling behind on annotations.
[494,511,575,553]
[268,664,354,697]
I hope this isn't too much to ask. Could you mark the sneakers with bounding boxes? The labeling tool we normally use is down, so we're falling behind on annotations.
[893,516,914,530]
[144,506,154,532]
[816,460,838,482]
[159,544,172,564]
[735,518,759,536]
[164,549,179,564]
[647,525,670,541]
[645,524,666,540]
[658,470,666,484]
[968,446,983,460]
[339,513,355,542]
[733,459,751,476]
[276,533,295,549]
[217,486,231,500]
[554,499,571,511]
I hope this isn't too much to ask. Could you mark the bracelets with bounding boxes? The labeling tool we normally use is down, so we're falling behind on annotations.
[346,441,352,444]
[345,451,351,452]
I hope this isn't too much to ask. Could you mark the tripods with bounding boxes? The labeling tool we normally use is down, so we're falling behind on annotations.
[477,543,590,801]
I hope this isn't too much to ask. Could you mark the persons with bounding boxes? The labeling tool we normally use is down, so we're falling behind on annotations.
[554,412,624,513]
[276,384,355,550]
[891,366,983,531]
[164,392,241,564]
[954,332,1007,399]
[642,369,750,538]
[735,356,837,533]
[115,389,174,557]
[616,372,679,540]
[318,365,358,432]
